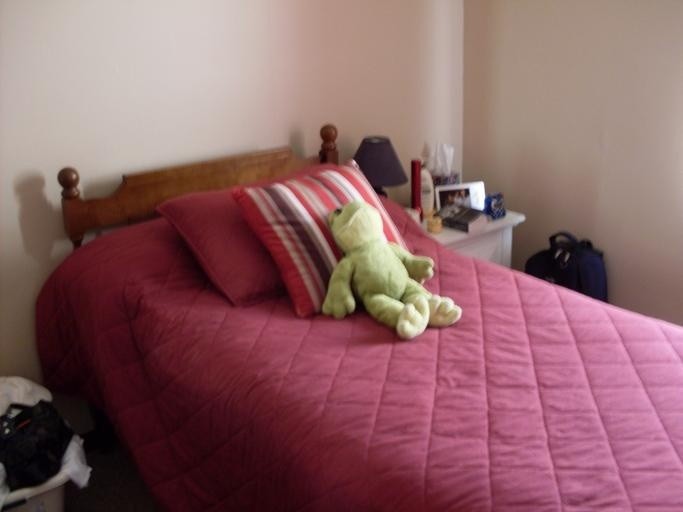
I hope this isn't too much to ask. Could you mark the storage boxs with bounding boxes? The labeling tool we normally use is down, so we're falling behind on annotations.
[2,439,80,512]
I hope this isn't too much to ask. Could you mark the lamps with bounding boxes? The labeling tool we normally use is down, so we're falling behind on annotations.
[354,136,409,202]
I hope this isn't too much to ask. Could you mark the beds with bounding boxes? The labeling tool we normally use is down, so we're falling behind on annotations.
[58,124,683,512]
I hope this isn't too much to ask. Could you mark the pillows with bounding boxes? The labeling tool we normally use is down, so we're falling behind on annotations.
[155,160,412,318]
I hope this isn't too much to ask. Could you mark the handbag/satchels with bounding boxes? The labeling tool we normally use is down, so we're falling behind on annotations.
[525,232,608,302]
[0,399,73,493]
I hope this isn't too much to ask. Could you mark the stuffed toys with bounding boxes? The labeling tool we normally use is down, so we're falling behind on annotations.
[321,201,462,340]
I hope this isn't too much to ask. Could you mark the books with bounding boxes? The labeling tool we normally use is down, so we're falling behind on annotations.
[433,207,486,233]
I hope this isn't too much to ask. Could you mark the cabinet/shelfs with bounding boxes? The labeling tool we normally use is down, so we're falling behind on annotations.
[404,205,527,269]
